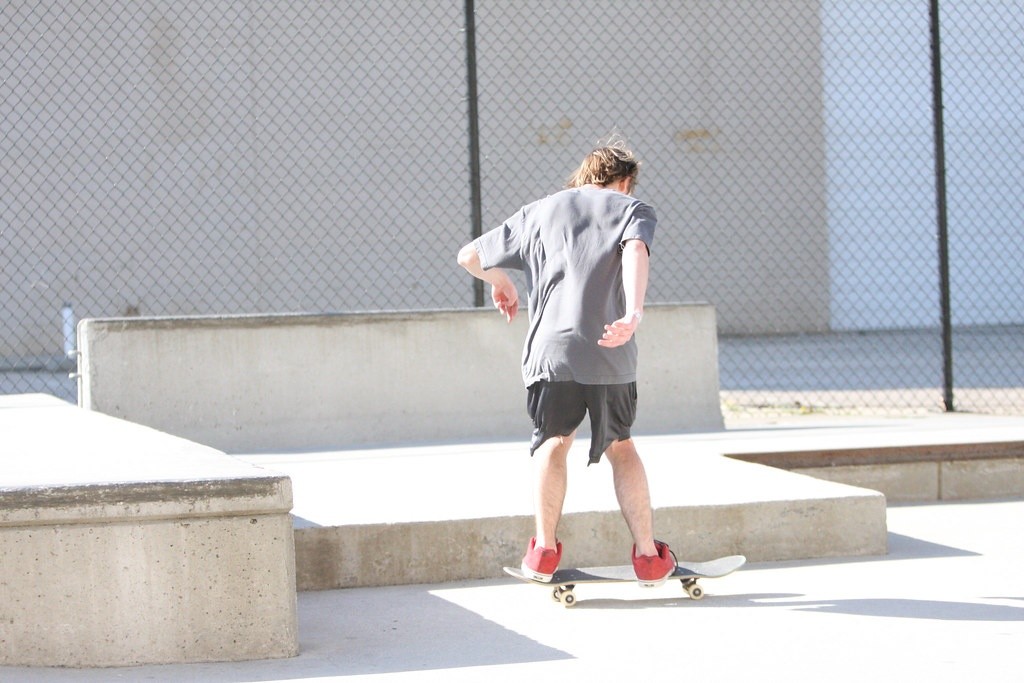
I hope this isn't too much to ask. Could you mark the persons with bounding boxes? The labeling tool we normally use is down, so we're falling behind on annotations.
[455,133,680,585]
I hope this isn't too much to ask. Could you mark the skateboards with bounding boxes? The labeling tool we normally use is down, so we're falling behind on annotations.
[503,553,748,607]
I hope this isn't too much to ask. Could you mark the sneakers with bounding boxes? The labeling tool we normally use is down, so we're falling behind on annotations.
[521,536,562,582]
[632,539,679,589]
